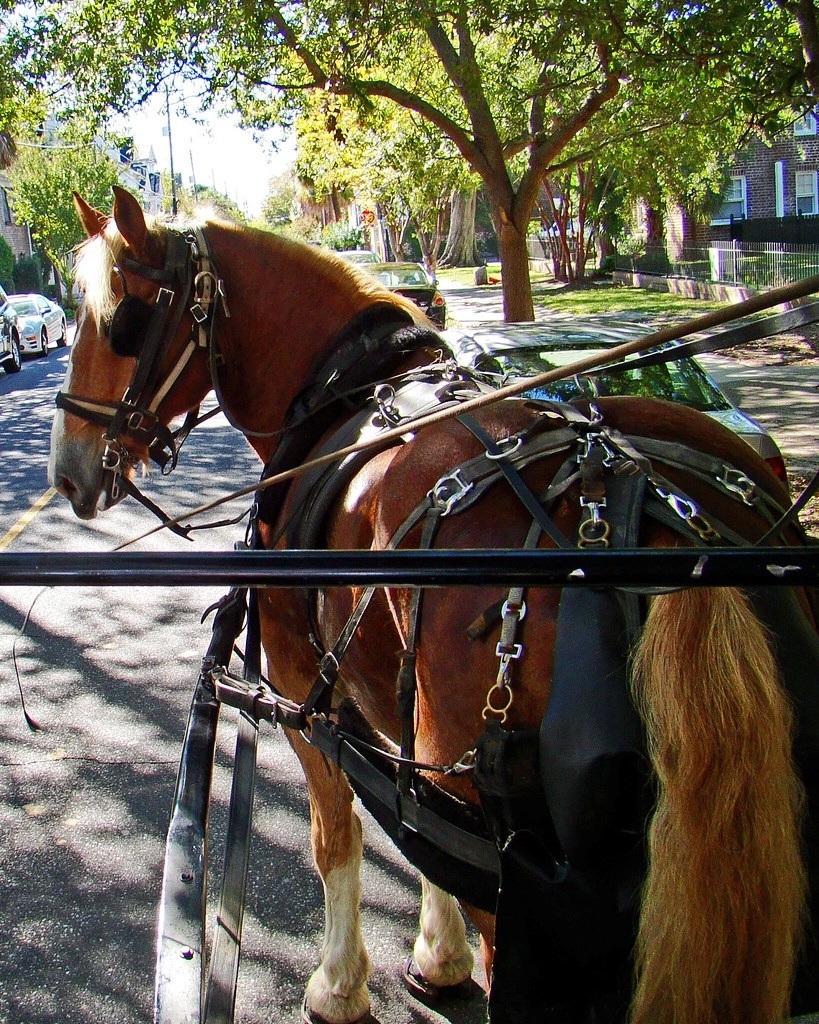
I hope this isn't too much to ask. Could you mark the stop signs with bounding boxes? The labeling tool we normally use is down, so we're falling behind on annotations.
[361,209,377,225]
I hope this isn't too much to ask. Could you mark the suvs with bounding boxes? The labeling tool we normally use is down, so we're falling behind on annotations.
[0,284,21,374]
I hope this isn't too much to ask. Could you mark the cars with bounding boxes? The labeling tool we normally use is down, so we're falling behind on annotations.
[355,261,447,332]
[441,316,791,517]
[338,249,382,274]
[8,293,67,358]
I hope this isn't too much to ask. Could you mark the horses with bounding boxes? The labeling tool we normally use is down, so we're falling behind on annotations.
[47,185,819,1023]
